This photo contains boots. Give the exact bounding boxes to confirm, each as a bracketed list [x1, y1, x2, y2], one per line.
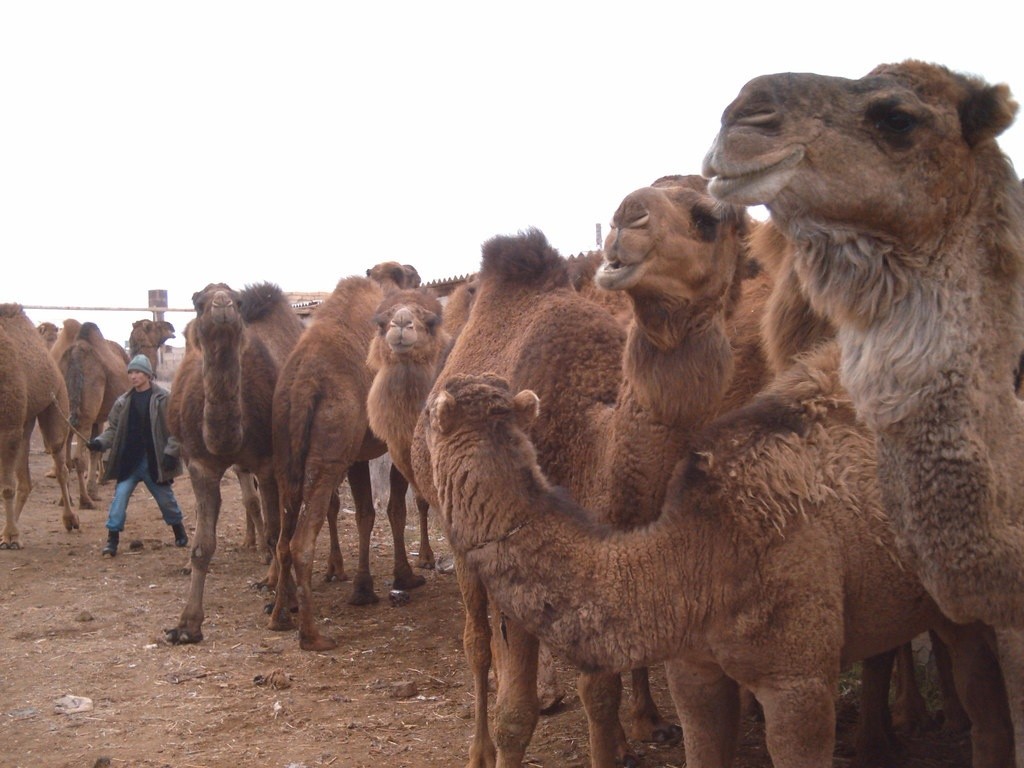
[172, 521, 188, 547]
[102, 529, 123, 557]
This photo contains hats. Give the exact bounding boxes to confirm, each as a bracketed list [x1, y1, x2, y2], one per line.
[128, 354, 153, 377]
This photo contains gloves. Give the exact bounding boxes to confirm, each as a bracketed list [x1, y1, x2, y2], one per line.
[161, 452, 177, 472]
[87, 438, 102, 451]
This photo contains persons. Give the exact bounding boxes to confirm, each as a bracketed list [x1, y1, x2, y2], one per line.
[86, 354, 188, 557]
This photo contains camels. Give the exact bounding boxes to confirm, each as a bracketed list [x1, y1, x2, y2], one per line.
[701, 57, 1024, 768]
[0, 303, 176, 552]
[411, 175, 1014, 768]
[162, 262, 480, 650]
